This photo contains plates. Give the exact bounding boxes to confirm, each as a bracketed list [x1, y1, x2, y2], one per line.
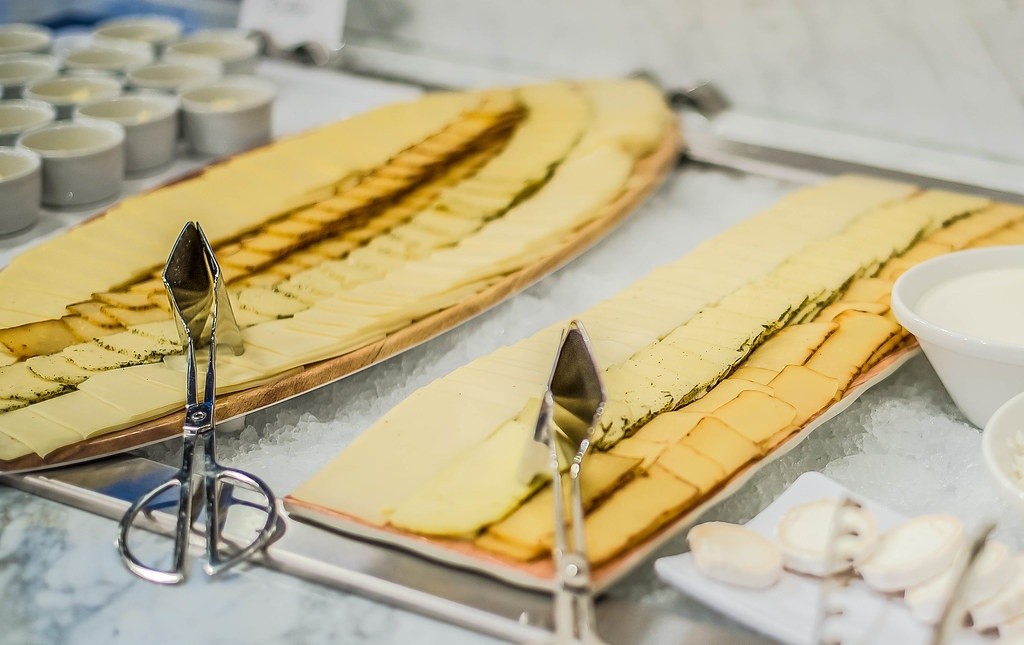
[655, 471, 1024, 645]
[284, 343, 922, 600]
[891, 245, 1024, 430]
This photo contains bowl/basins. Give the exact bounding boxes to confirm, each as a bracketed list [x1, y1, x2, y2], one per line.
[982, 391, 1024, 501]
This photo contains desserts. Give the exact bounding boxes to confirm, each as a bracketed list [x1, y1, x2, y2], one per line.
[687, 497, 1024, 645]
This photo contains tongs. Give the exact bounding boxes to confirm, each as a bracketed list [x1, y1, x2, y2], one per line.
[534, 318, 607, 645]
[116, 221, 279, 586]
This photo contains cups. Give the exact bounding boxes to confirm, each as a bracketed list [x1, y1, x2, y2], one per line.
[125, 57, 224, 95]
[0, 146, 43, 236]
[176, 76, 275, 158]
[70, 91, 179, 171]
[164, 31, 266, 76]
[21, 70, 123, 119]
[0, 113, 683, 472]
[15, 119, 127, 206]
[0, 24, 53, 59]
[0, 98, 57, 145]
[88, 17, 181, 65]
[0, 59, 63, 99]
[63, 36, 126, 81]
